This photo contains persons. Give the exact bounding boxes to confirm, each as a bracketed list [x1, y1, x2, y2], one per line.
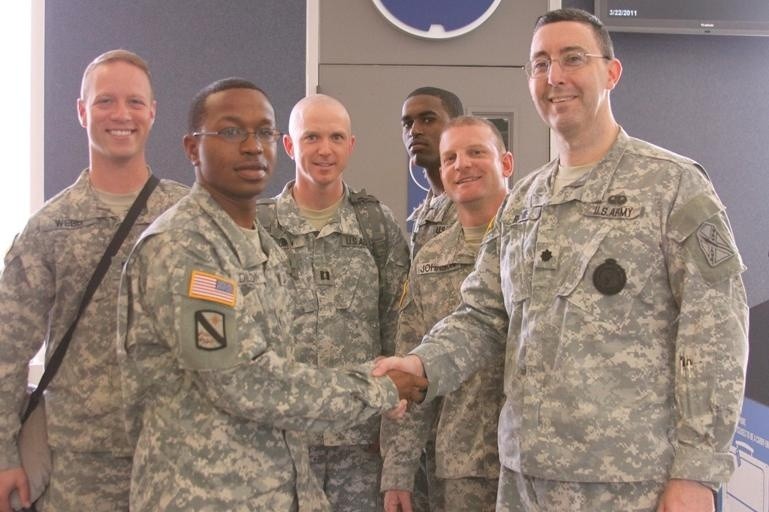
[378, 114, 515, 512]
[119, 74, 430, 511]
[255, 91, 416, 512]
[399, 84, 466, 264]
[1, 48, 191, 512]
[372, 6, 750, 512]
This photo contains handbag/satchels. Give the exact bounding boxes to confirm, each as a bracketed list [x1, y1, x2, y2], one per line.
[8, 387, 53, 512]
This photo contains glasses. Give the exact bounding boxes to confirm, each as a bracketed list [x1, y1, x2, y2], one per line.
[192, 127, 283, 145]
[521, 50, 611, 78]
